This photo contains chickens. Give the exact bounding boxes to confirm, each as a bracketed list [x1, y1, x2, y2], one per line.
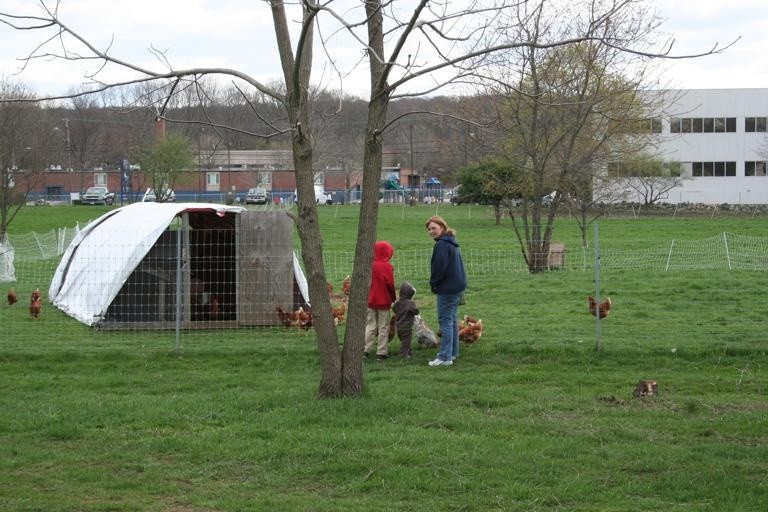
[457, 315, 483, 344]
[29, 287, 41, 318]
[326, 281, 334, 297]
[376, 314, 397, 344]
[341, 274, 352, 296]
[7, 287, 18, 305]
[587, 295, 613, 319]
[414, 312, 439, 347]
[332, 303, 346, 320]
[273, 306, 313, 337]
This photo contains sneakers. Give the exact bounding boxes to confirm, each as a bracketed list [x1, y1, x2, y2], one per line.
[363, 351, 369, 359]
[428, 357, 453, 367]
[376, 354, 389, 361]
[436, 352, 457, 361]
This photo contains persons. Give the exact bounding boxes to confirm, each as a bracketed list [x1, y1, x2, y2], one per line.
[208, 189, 459, 208]
[392, 280, 419, 358]
[424, 215, 468, 366]
[363, 242, 396, 360]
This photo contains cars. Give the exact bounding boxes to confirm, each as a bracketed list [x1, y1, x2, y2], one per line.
[146, 187, 175, 202]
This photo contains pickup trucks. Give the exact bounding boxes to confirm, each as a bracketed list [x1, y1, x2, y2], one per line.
[246, 187, 271, 203]
[83, 186, 116, 205]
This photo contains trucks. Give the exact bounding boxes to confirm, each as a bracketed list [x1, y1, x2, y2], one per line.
[294, 184, 332, 205]
